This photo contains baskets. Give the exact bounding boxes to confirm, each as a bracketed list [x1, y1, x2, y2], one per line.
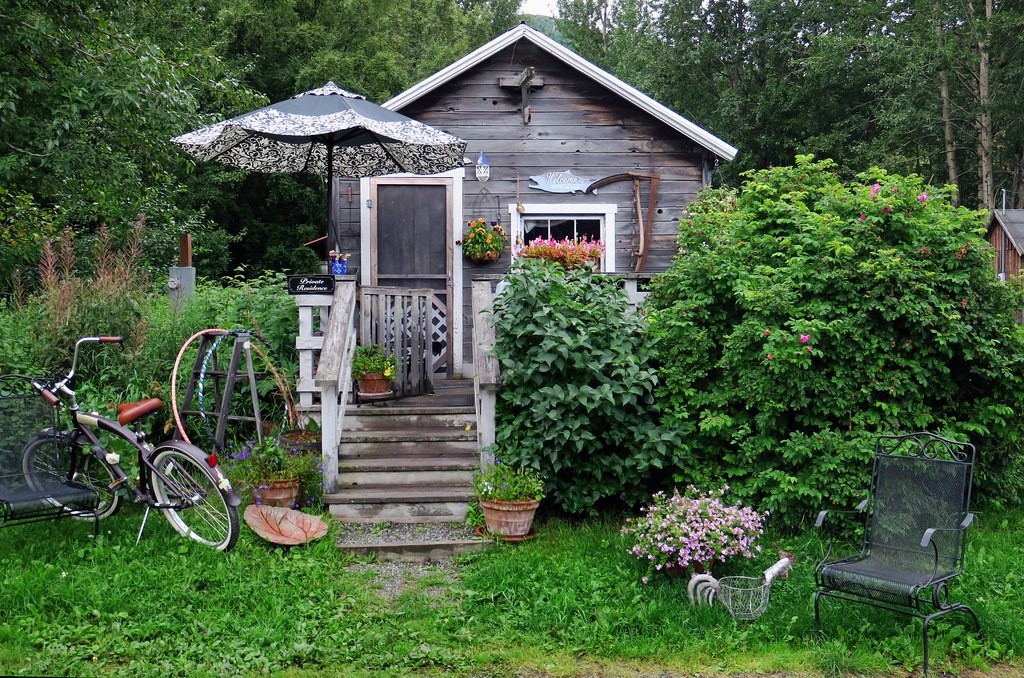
[470, 251, 500, 262]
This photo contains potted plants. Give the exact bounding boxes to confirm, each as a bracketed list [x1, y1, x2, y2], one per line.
[470, 461, 545, 542]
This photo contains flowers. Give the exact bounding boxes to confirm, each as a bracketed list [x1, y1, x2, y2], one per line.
[455, 217, 509, 261]
[225, 436, 328, 507]
[352, 344, 403, 382]
[620, 484, 771, 584]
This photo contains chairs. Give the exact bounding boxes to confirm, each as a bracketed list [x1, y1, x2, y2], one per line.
[814, 432, 985, 678]
[0, 374, 100, 548]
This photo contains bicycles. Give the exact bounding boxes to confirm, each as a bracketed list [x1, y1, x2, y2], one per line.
[20, 336, 242, 554]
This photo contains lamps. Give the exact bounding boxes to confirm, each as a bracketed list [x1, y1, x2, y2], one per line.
[475, 150, 490, 182]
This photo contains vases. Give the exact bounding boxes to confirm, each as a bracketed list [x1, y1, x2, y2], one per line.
[356, 373, 393, 399]
[247, 478, 299, 509]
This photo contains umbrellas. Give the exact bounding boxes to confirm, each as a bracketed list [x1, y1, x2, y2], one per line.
[169, 81, 474, 273]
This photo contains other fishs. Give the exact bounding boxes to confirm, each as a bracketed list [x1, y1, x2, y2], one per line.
[528, 169, 598, 195]
